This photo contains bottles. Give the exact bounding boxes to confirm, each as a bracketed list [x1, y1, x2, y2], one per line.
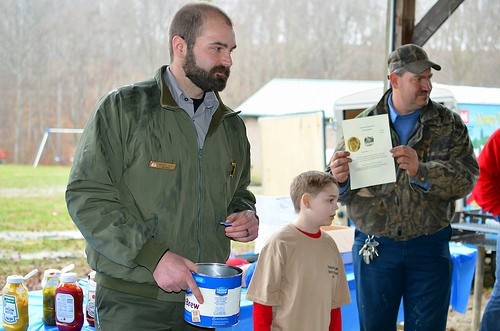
[54, 272, 84, 331]
[85, 270, 97, 326]
[42, 268, 62, 326]
[2, 274, 29, 331]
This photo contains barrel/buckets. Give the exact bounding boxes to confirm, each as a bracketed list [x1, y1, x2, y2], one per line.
[184, 262, 243, 329]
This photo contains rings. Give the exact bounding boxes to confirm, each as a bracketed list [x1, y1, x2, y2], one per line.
[245, 229, 249, 238]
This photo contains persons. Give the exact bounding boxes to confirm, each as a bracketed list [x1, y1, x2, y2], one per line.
[473, 128, 500, 331]
[65, 3, 257, 331]
[325, 41, 478, 331]
[245, 171, 352, 331]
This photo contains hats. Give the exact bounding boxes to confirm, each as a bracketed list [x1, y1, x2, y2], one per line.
[387, 44, 441, 75]
